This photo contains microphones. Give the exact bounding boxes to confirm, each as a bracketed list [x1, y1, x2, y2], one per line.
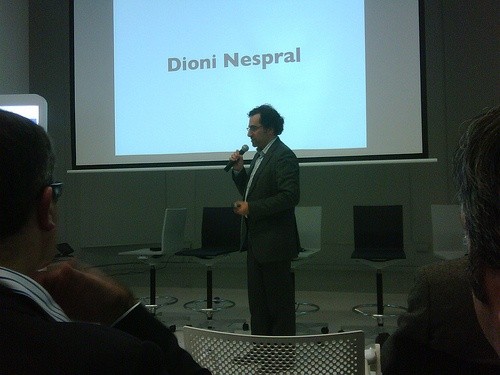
[224, 145, 249, 172]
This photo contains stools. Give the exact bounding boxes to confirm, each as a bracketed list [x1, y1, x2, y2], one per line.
[170, 205, 248, 333]
[431, 204, 467, 264]
[115, 206, 187, 319]
[350, 205, 407, 330]
[284, 205, 323, 315]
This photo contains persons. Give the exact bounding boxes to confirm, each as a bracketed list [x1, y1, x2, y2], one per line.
[232, 105, 301, 373]
[0, 109, 212, 375]
[381, 104, 500, 375]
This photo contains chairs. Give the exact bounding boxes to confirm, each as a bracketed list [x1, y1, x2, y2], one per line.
[181, 326, 370, 374]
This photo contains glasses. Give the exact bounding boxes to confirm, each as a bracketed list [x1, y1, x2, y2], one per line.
[247, 125, 262, 132]
[34, 181, 65, 203]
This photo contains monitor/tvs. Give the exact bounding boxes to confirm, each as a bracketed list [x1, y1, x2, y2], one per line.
[0, 94, 48, 132]
